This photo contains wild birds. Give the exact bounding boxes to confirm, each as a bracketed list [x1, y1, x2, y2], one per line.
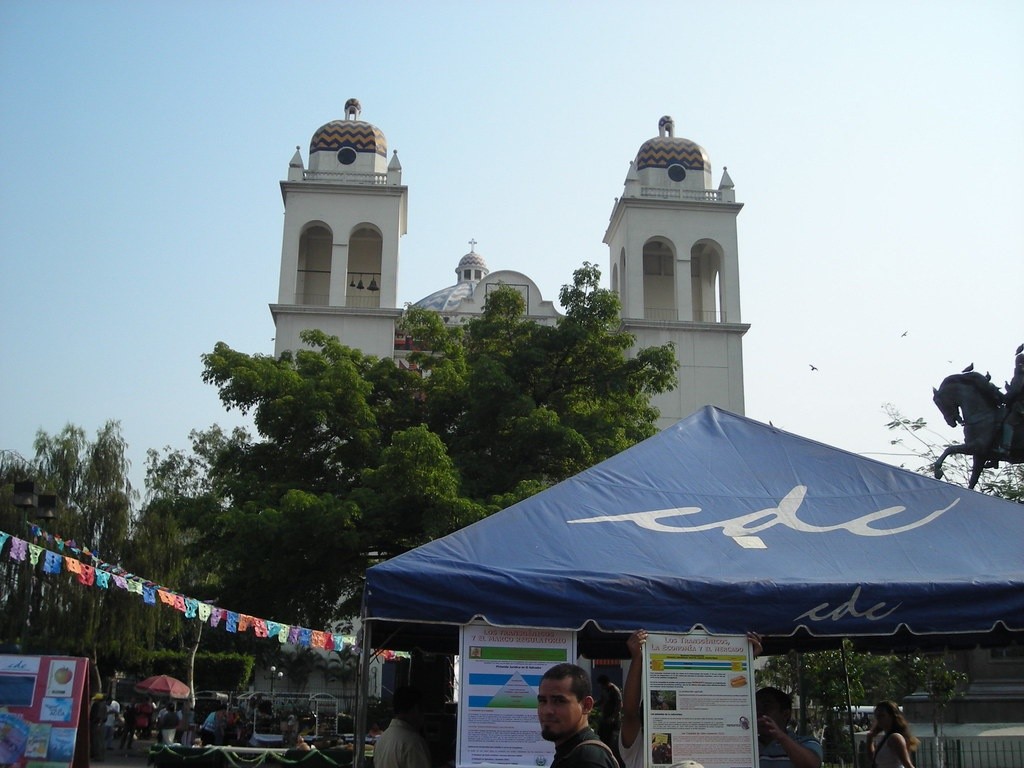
[985, 370, 991, 382]
[961, 362, 974, 373]
[808, 364, 818, 372]
[901, 330, 908, 338]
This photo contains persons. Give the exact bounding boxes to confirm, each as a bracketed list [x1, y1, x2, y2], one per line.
[756, 686, 824, 768]
[90, 692, 391, 762]
[619, 629, 762, 768]
[991, 344, 1024, 457]
[788, 710, 872, 733]
[538, 663, 618, 768]
[373, 686, 432, 768]
[866, 701, 921, 768]
[592, 676, 623, 746]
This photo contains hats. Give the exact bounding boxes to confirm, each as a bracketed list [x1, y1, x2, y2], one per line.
[92, 693, 104, 700]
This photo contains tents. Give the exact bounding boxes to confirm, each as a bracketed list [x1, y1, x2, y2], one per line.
[352, 404, 1024, 768]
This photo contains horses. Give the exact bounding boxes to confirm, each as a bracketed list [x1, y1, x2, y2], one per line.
[932, 372, 1024, 490]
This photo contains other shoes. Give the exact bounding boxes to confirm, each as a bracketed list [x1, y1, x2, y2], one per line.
[107, 747, 114, 750]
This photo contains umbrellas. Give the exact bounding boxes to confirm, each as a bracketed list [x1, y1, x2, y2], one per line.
[137, 674, 190, 698]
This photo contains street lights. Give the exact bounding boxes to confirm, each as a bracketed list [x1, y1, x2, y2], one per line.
[264, 666, 284, 698]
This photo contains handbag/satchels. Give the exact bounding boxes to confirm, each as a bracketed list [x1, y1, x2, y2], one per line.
[137, 714, 148, 727]
